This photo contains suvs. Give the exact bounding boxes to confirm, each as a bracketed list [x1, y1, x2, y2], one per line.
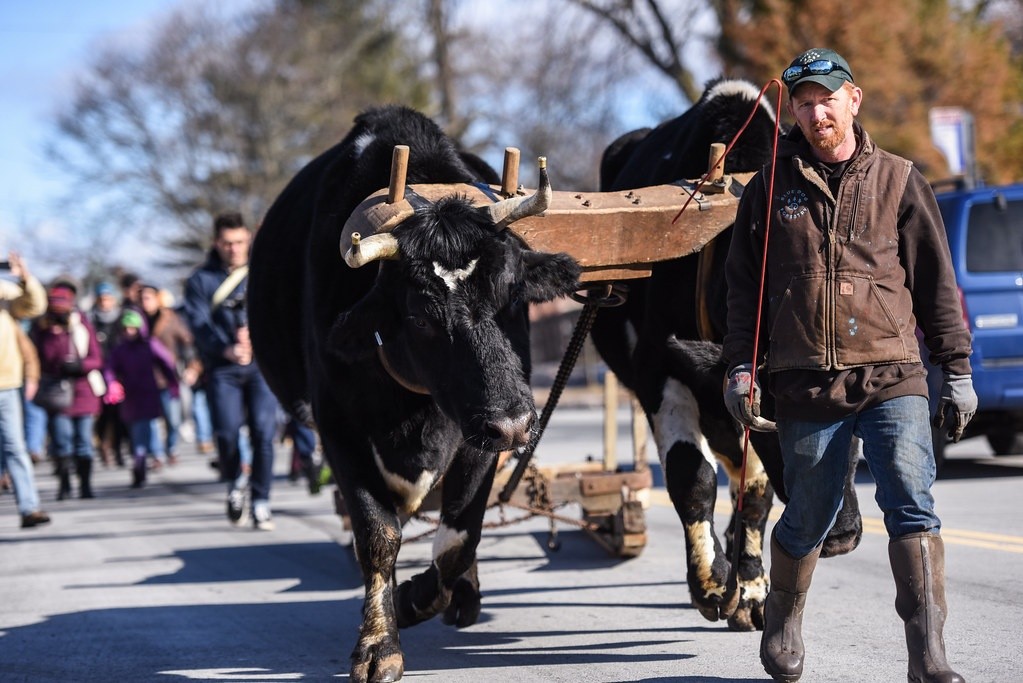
[928, 179, 1023, 457]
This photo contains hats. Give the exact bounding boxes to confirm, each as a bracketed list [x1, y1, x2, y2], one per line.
[787, 48, 854, 100]
[120, 309, 142, 328]
[137, 279, 163, 290]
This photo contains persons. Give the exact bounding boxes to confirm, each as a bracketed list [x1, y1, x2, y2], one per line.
[184, 211, 278, 531]
[719, 47, 978, 683]
[0, 251, 332, 528]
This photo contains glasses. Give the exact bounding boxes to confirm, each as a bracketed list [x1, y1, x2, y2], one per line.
[780, 59, 838, 82]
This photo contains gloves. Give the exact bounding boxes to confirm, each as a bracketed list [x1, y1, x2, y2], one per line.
[721, 363, 779, 432]
[933, 370, 978, 444]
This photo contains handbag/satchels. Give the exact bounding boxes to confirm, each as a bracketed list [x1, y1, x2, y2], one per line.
[30, 378, 74, 410]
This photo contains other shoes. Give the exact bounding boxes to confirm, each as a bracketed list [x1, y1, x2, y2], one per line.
[226, 488, 246, 522]
[131, 468, 147, 489]
[19, 511, 50, 526]
[250, 498, 277, 530]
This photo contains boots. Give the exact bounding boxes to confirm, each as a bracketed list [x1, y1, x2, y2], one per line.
[888, 532, 967, 683]
[74, 456, 96, 499]
[54, 465, 72, 501]
[759, 525, 824, 683]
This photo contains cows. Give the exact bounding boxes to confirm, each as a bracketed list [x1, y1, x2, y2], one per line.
[580, 73, 863, 635]
[244, 103, 585, 683]
